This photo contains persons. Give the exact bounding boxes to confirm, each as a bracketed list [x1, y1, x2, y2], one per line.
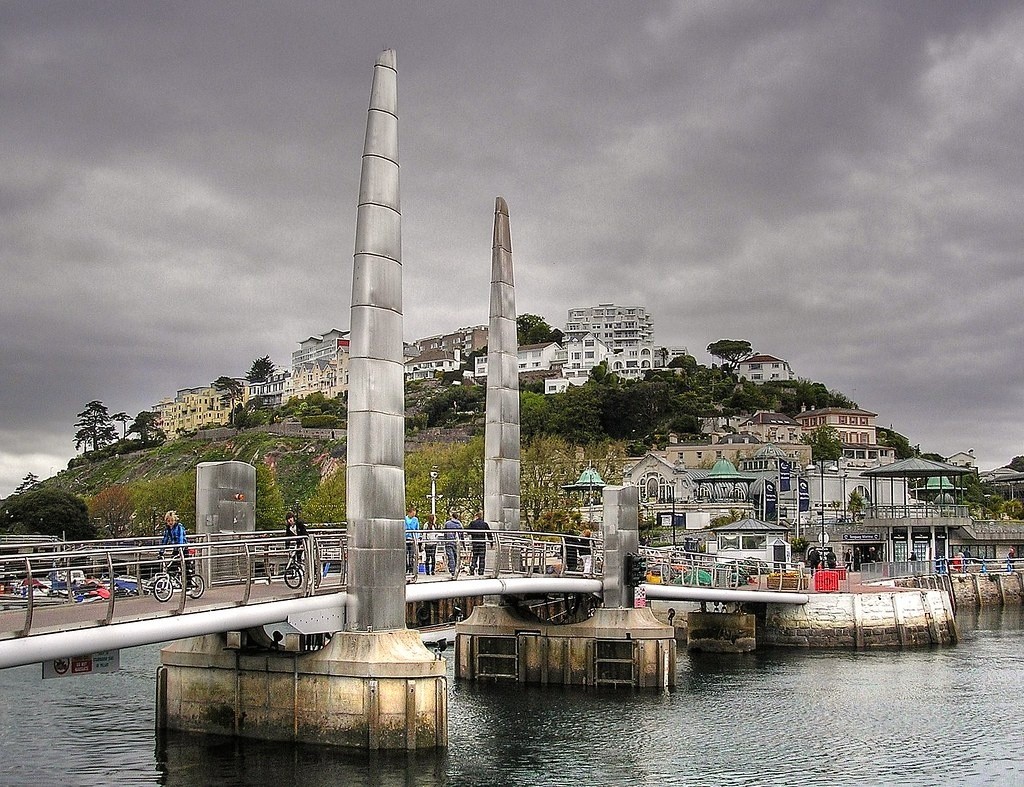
[958, 547, 971, 564]
[1009, 545, 1016, 569]
[827, 548, 836, 569]
[285, 512, 310, 564]
[467, 513, 493, 575]
[845, 549, 853, 572]
[405, 508, 420, 572]
[559, 529, 591, 571]
[445, 512, 466, 575]
[807, 547, 820, 577]
[157, 510, 192, 595]
[422, 515, 435, 575]
[908, 552, 916, 561]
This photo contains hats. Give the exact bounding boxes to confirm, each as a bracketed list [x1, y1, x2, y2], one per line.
[910, 550, 914, 552]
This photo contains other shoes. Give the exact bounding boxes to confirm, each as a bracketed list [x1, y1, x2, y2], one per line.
[186, 588, 193, 595]
[173, 580, 181, 588]
[426, 572, 435, 575]
[295, 577, 299, 580]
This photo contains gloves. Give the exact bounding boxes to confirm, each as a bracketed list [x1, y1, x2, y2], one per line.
[285, 547, 290, 553]
[157, 552, 164, 559]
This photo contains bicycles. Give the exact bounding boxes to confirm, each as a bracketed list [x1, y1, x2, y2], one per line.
[284, 544, 321, 589]
[154, 554, 205, 602]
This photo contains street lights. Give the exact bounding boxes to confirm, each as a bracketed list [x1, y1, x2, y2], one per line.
[587, 459, 597, 531]
[805, 453, 839, 568]
[669, 479, 676, 545]
[429, 466, 439, 524]
